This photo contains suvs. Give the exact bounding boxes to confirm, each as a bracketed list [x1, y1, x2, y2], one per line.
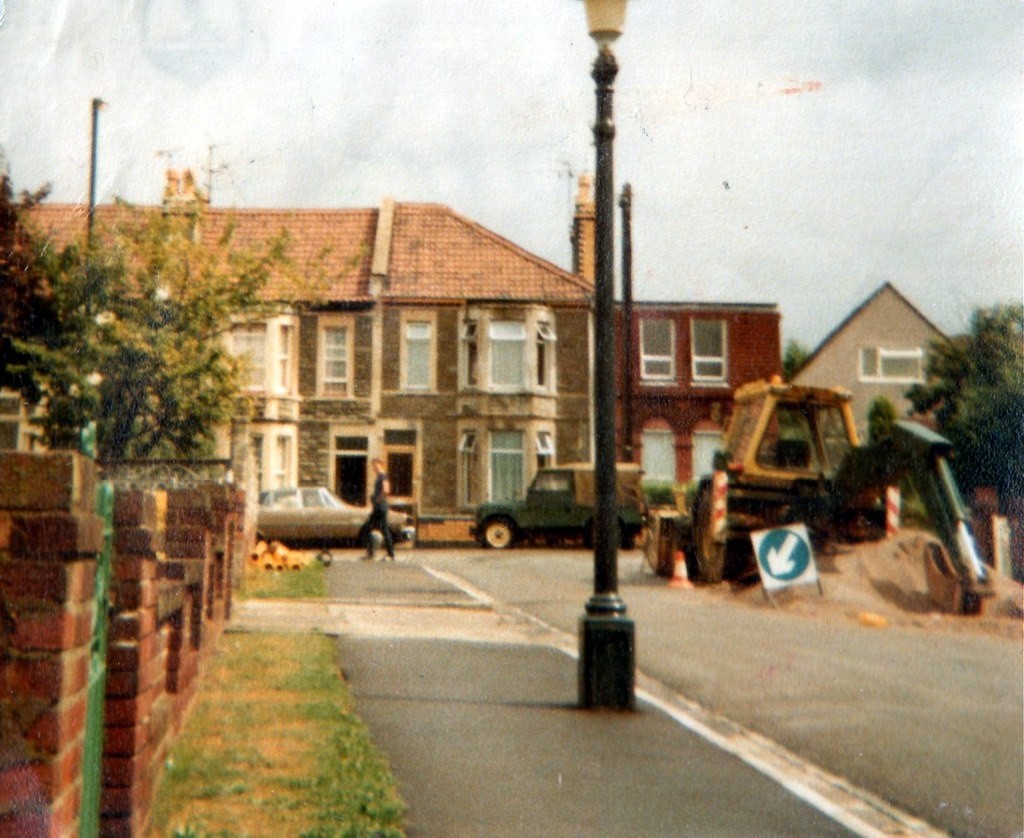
[475, 464, 648, 548]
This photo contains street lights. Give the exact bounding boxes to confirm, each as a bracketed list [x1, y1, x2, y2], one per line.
[87, 97, 107, 247]
[577, 1, 637, 717]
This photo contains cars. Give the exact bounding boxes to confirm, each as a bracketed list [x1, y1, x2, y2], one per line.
[257, 485, 415, 548]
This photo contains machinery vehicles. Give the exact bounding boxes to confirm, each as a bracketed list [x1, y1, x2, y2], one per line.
[644, 375, 1000, 615]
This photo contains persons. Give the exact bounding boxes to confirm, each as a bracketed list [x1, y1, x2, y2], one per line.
[357, 459, 396, 563]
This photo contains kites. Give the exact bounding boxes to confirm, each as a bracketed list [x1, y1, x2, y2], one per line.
[775, 77, 827, 95]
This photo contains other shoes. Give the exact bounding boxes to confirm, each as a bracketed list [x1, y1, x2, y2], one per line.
[359, 554, 375, 561]
[379, 554, 395, 561]
